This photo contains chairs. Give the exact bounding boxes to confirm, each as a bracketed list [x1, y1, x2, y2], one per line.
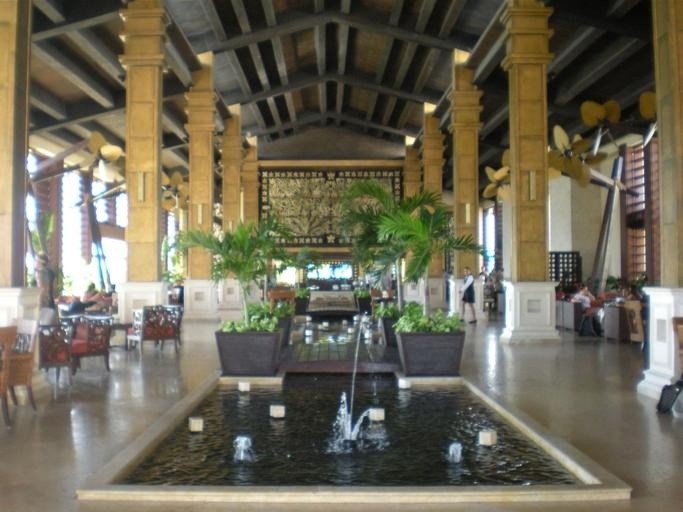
[0, 294, 115, 429]
[556, 290, 648, 351]
[127, 285, 184, 354]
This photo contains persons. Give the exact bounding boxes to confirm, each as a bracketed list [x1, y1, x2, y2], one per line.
[491, 269, 503, 313]
[622, 288, 629, 297]
[627, 285, 641, 300]
[459, 266, 476, 320]
[478, 267, 487, 278]
[569, 284, 591, 335]
[58, 279, 116, 314]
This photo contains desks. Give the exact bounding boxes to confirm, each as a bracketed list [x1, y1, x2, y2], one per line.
[110, 323, 132, 351]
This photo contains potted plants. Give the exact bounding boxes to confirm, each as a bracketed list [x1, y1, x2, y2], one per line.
[336, 178, 498, 375]
[169, 269, 186, 286]
[165, 209, 323, 375]
